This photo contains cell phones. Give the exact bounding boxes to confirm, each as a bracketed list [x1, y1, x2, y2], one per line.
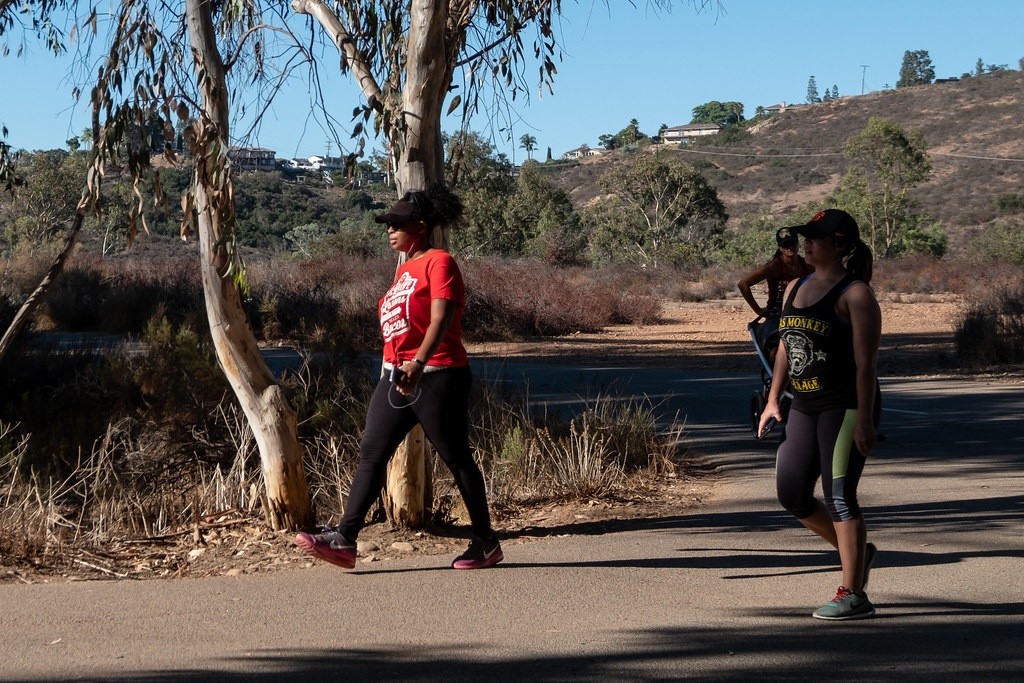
[758, 418, 776, 438]
[390, 366, 418, 397]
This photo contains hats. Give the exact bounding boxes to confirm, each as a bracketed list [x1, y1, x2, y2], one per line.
[375, 198, 428, 227]
[776, 226, 799, 246]
[788, 209, 860, 246]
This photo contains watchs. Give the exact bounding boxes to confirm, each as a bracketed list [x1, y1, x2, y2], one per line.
[412, 357, 424, 366]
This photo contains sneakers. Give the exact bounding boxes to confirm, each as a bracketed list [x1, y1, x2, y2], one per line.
[452, 529, 504, 569]
[296, 527, 356, 569]
[863, 542, 877, 589]
[813, 587, 876, 620]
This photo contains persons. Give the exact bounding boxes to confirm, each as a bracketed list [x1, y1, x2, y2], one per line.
[292, 201, 504, 569]
[739, 209, 882, 621]
